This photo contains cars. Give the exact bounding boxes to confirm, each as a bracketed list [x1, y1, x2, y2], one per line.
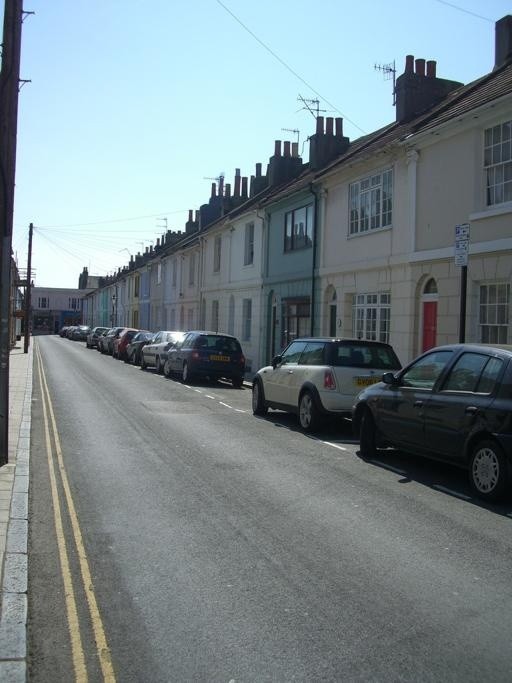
[252, 336, 403, 432]
[60, 325, 246, 387]
[352, 341, 511, 505]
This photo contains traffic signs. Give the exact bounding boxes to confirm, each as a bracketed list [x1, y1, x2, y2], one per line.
[455, 222, 470, 267]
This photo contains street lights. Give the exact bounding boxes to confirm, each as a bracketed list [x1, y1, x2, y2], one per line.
[111, 293, 116, 327]
